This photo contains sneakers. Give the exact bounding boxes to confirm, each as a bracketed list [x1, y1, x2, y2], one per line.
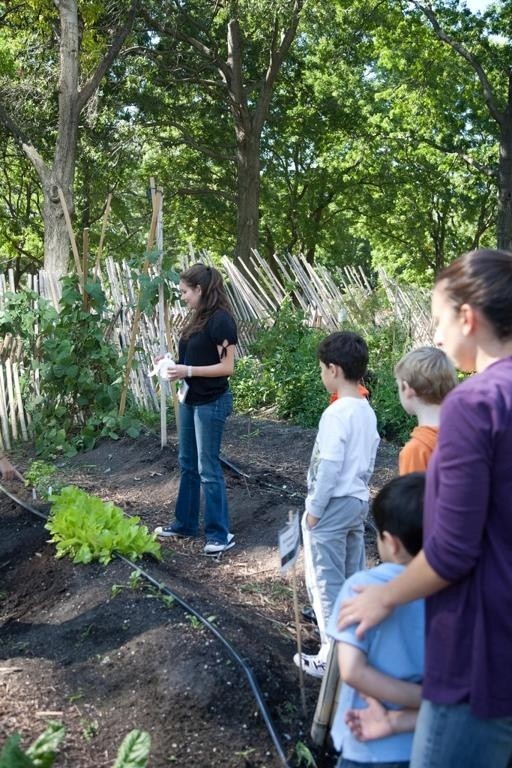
[293, 653, 328, 679]
[155, 526, 192, 538]
[301, 607, 317, 622]
[204, 540, 236, 552]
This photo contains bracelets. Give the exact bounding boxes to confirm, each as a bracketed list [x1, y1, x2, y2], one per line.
[188, 365, 192, 377]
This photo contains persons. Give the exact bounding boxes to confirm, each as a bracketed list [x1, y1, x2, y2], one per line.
[335, 249, 511, 768]
[391, 345, 458, 477]
[291, 330, 382, 683]
[320, 469, 425, 768]
[152, 262, 240, 555]
